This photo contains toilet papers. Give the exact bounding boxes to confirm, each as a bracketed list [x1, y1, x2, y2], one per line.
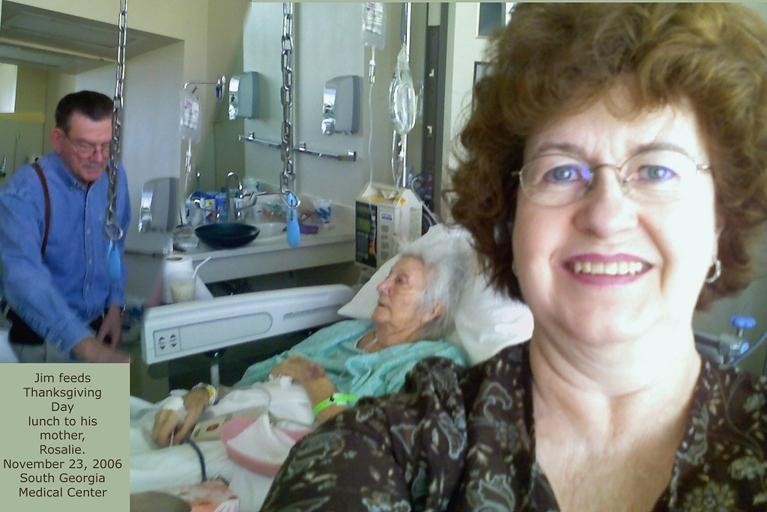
[162, 255, 193, 305]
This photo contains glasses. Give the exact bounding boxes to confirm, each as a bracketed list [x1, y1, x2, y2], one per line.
[65, 132, 111, 156]
[504, 147, 711, 204]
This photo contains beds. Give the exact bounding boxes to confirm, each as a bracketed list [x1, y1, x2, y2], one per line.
[131, 330, 740, 512]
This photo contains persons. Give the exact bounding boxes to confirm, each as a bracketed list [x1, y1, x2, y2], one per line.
[258, 2, 766, 511]
[151, 224, 479, 448]
[0, 91, 136, 363]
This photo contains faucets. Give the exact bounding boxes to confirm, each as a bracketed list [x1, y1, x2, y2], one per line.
[225, 170, 258, 221]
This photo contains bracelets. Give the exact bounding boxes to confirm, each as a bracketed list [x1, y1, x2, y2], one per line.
[312, 395, 356, 416]
[191, 382, 216, 406]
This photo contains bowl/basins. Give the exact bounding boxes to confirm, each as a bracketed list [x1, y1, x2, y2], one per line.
[194, 222, 287, 248]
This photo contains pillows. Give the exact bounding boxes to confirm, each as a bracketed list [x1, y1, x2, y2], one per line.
[335, 221, 535, 370]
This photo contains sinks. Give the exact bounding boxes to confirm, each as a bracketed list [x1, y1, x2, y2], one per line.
[248, 221, 289, 243]
